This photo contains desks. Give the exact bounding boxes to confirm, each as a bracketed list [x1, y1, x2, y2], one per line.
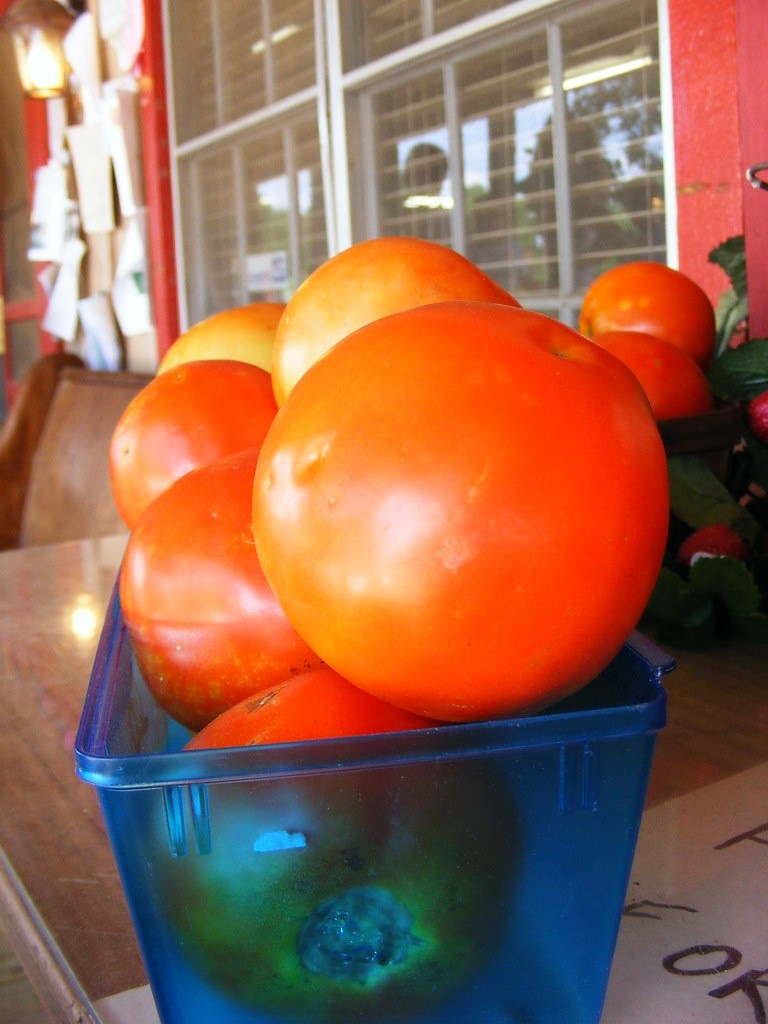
[0, 534, 768, 1024]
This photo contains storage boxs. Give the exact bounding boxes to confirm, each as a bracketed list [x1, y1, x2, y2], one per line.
[71, 591, 674, 1024]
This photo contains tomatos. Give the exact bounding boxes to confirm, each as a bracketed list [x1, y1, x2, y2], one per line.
[106, 237, 768, 1024]
